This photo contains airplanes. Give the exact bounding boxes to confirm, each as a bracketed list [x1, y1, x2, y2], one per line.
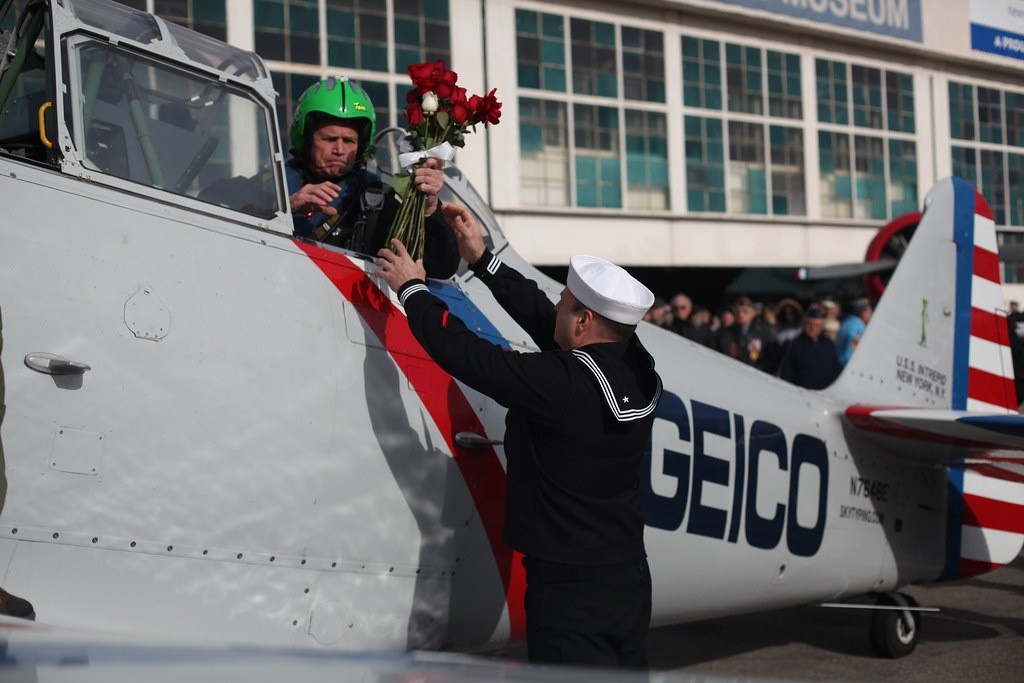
[2, 3, 1023, 680]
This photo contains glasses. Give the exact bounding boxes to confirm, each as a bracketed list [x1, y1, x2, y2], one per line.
[674, 306, 685, 310]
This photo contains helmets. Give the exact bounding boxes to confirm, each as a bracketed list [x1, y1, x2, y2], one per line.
[291, 77, 376, 166]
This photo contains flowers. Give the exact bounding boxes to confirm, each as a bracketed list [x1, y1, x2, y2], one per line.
[382, 58, 504, 259]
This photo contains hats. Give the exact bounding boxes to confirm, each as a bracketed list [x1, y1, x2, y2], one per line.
[736, 297, 754, 308]
[857, 300, 869, 309]
[1009, 298, 1020, 302]
[567, 254, 655, 325]
[807, 309, 826, 318]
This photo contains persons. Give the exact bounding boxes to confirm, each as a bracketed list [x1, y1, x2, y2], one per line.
[376, 204, 664, 683]
[1006, 301, 1024, 407]
[197, 75, 460, 281]
[642, 292, 871, 391]
